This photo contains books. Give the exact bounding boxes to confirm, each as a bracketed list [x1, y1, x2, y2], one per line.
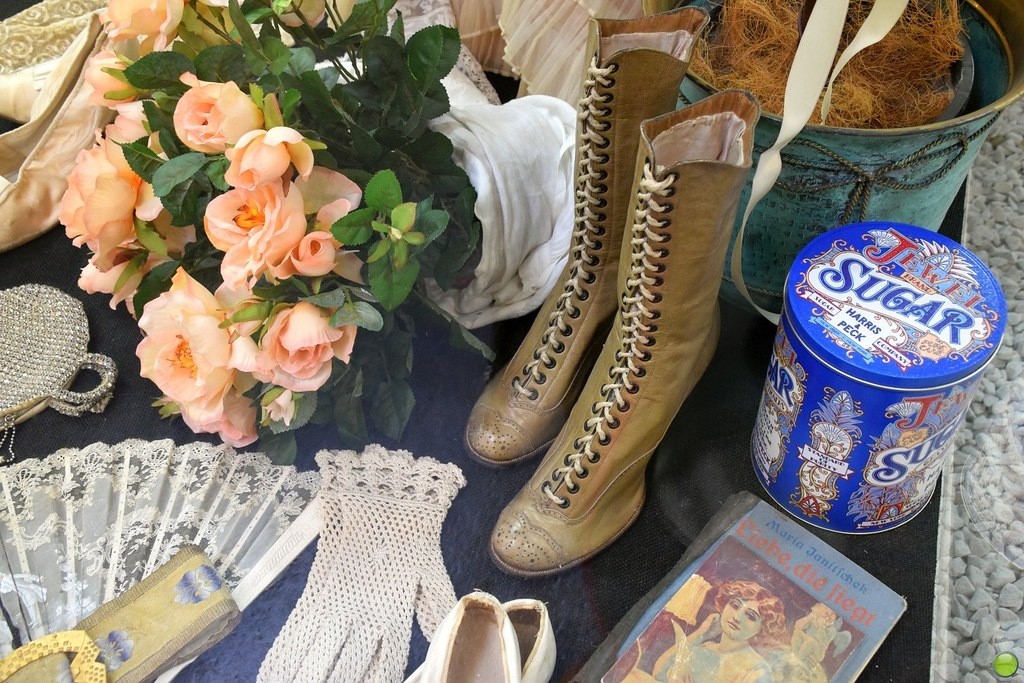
[567, 490, 907, 683]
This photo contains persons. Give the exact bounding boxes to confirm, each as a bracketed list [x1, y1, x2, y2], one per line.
[654, 578, 836, 683]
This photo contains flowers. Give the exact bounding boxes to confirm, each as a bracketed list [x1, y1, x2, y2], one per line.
[59, 0, 497, 466]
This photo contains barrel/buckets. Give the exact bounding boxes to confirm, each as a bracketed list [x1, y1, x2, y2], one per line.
[642, 0, 1024, 316]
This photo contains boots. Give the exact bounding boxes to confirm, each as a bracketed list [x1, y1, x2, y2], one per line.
[464, 6, 710, 471]
[489, 89, 761, 577]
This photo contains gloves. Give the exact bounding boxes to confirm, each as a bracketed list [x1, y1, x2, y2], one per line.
[257, 444, 468, 683]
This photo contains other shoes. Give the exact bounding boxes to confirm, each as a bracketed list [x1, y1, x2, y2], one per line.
[0, 6, 140, 251]
[403, 589, 556, 683]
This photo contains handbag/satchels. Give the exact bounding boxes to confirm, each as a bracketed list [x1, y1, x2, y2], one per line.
[0, 284, 118, 432]
